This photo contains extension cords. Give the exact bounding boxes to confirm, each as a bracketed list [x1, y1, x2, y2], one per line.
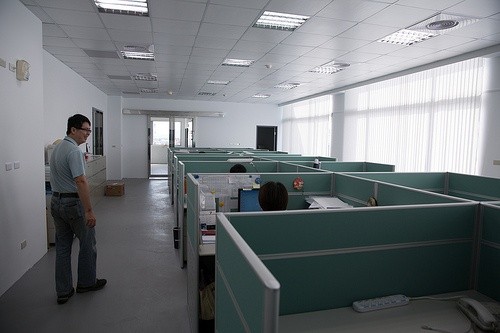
[352, 294, 409, 313]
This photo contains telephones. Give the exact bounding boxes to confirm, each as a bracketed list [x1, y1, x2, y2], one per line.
[86, 143, 91, 152]
[456, 297, 500, 331]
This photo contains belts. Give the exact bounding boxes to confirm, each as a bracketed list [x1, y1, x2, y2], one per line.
[53, 191, 79, 197]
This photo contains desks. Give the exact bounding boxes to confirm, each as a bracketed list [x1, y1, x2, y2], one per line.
[200, 229, 216, 255]
[46, 155, 107, 243]
[278, 289, 500, 333]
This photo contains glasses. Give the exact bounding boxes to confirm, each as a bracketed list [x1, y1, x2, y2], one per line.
[80, 127, 91, 132]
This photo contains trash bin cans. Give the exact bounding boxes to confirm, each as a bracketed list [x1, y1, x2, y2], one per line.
[173, 226, 180, 249]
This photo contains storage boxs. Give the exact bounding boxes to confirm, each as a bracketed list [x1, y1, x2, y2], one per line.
[104, 183, 125, 197]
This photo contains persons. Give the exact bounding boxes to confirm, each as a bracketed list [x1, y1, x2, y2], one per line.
[51, 114, 108, 305]
[229, 164, 247, 173]
[258, 181, 289, 212]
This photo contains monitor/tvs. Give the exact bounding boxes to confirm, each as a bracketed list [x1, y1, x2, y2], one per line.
[238, 188, 263, 212]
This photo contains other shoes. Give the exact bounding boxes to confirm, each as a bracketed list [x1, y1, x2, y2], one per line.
[76, 278, 107, 293]
[57, 287, 74, 304]
